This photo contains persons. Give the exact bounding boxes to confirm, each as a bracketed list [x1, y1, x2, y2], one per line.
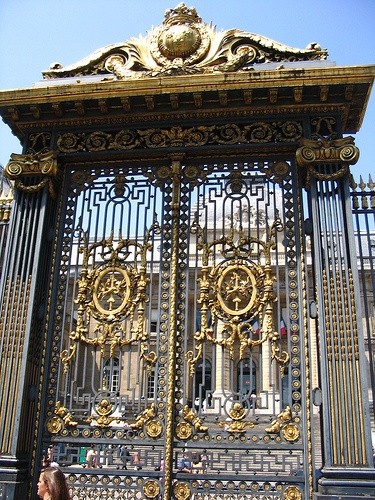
[45, 443, 207, 475]
[36, 466, 72, 500]
